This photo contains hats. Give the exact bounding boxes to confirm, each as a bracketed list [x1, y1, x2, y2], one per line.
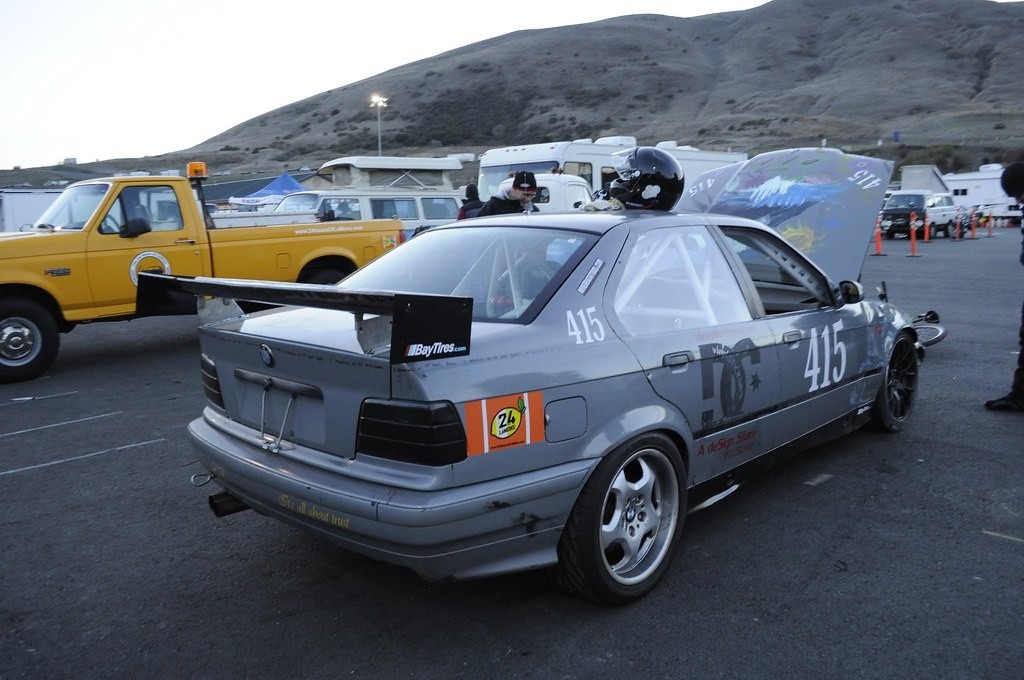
[466, 184, 479, 197]
[513, 171, 538, 192]
[1001, 163, 1024, 197]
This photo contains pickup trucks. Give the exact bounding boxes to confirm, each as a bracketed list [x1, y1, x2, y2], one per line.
[0, 173, 408, 385]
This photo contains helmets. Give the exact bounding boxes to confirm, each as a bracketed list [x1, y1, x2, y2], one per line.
[611, 146, 685, 210]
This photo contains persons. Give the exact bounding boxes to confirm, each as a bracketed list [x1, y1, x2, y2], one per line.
[457, 184, 485, 221]
[476, 171, 540, 218]
[983, 162, 1024, 414]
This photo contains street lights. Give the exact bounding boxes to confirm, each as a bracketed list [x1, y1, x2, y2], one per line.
[369, 95, 389, 156]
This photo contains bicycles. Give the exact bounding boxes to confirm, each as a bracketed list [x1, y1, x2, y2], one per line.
[873, 280, 947, 347]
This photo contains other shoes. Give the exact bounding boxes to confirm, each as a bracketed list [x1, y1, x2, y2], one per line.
[985, 395, 1024, 412]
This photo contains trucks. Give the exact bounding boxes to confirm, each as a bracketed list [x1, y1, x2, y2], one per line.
[476, 134, 749, 207]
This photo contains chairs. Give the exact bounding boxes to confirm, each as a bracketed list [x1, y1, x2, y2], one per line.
[325, 199, 451, 220]
[135, 204, 152, 223]
[521, 261, 566, 303]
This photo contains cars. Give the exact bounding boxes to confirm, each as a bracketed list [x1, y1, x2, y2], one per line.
[134, 145, 925, 610]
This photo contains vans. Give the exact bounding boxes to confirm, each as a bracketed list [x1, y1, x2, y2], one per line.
[872, 181, 969, 241]
[217, 134, 596, 245]
